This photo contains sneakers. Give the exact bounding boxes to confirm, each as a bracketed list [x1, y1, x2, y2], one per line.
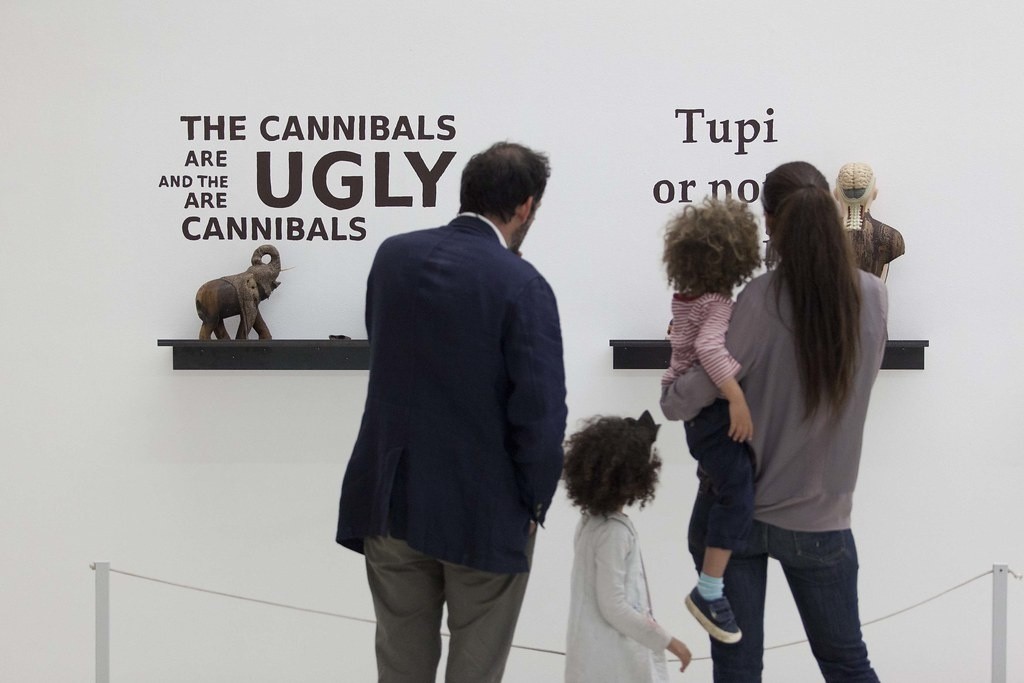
[685, 586, 743, 645]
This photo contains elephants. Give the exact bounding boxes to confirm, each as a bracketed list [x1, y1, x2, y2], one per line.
[195, 245, 281, 340]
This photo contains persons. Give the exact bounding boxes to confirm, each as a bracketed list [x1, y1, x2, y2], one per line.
[560, 413, 691, 683]
[658, 159, 880, 683]
[334, 141, 568, 683]
[831, 162, 905, 286]
[660, 194, 757, 644]
